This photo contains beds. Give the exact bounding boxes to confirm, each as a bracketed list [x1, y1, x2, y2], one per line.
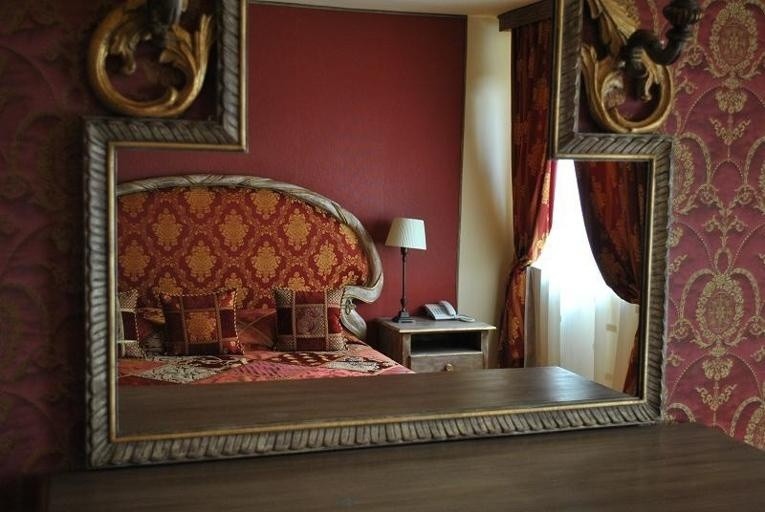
[114, 172, 416, 387]
[117, 288, 148, 360]
[158, 288, 246, 357]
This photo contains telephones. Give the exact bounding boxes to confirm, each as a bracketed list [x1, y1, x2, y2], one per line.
[425, 300, 475, 322]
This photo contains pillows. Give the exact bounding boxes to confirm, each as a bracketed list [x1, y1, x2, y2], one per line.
[272, 286, 349, 352]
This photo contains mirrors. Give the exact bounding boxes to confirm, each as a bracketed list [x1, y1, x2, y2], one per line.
[82, 0, 676, 468]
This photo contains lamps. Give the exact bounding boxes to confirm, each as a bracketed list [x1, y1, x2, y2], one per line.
[385, 216, 427, 324]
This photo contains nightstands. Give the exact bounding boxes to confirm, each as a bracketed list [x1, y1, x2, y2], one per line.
[377, 315, 497, 374]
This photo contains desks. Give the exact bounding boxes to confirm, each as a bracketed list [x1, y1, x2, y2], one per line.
[40, 421, 765, 512]
